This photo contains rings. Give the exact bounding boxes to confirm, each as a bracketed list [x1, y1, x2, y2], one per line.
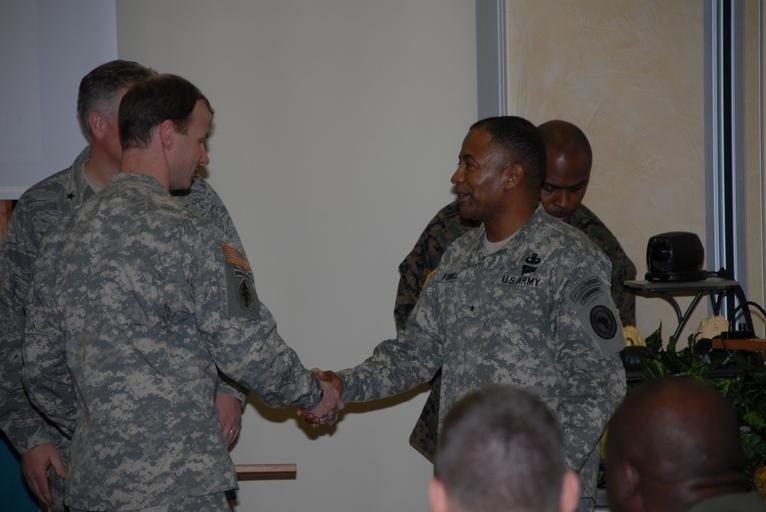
[318, 416, 328, 425]
[229, 429, 236, 434]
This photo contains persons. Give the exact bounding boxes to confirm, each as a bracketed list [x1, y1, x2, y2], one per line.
[602, 375, 766, 512]
[393, 118, 638, 472]
[20, 72, 346, 512]
[0, 57, 259, 512]
[295, 113, 629, 512]
[426, 384, 583, 512]
